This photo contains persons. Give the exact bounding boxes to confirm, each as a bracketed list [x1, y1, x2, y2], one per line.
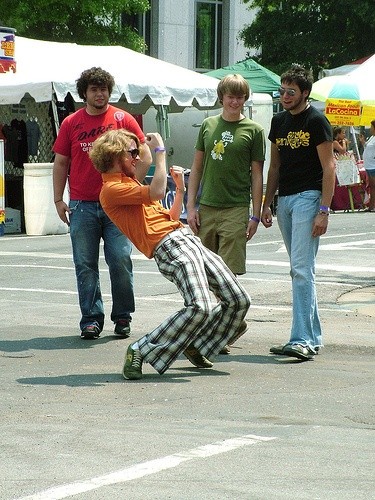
[333, 127, 348, 154]
[186, 74, 266, 354]
[359, 121, 375, 212]
[89, 128, 251, 380]
[261, 67, 336, 359]
[53, 66, 152, 338]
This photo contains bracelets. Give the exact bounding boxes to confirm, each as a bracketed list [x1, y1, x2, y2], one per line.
[318, 206, 330, 216]
[154, 147, 165, 152]
[251, 217, 259, 223]
[176, 187, 186, 193]
[55, 200, 62, 204]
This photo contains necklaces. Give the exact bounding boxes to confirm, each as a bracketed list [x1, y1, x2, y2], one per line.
[302, 104, 308, 111]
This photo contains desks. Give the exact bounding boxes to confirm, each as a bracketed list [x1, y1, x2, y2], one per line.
[145, 175, 202, 213]
[330, 170, 370, 213]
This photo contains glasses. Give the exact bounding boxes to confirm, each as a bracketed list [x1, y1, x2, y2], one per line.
[127, 149, 139, 158]
[278, 87, 301, 96]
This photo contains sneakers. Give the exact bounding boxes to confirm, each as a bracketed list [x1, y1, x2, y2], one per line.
[227, 320, 249, 345]
[269, 344, 284, 354]
[122, 345, 143, 381]
[283, 343, 314, 361]
[81, 326, 100, 339]
[184, 344, 214, 368]
[114, 321, 131, 337]
[219, 345, 230, 354]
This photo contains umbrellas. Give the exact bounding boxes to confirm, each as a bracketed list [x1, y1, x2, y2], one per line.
[308, 74, 375, 159]
[204, 59, 281, 120]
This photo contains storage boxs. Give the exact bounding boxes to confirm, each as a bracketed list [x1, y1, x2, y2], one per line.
[4, 207, 21, 233]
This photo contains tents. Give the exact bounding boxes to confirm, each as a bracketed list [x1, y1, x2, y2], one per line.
[0, 36, 254, 140]
